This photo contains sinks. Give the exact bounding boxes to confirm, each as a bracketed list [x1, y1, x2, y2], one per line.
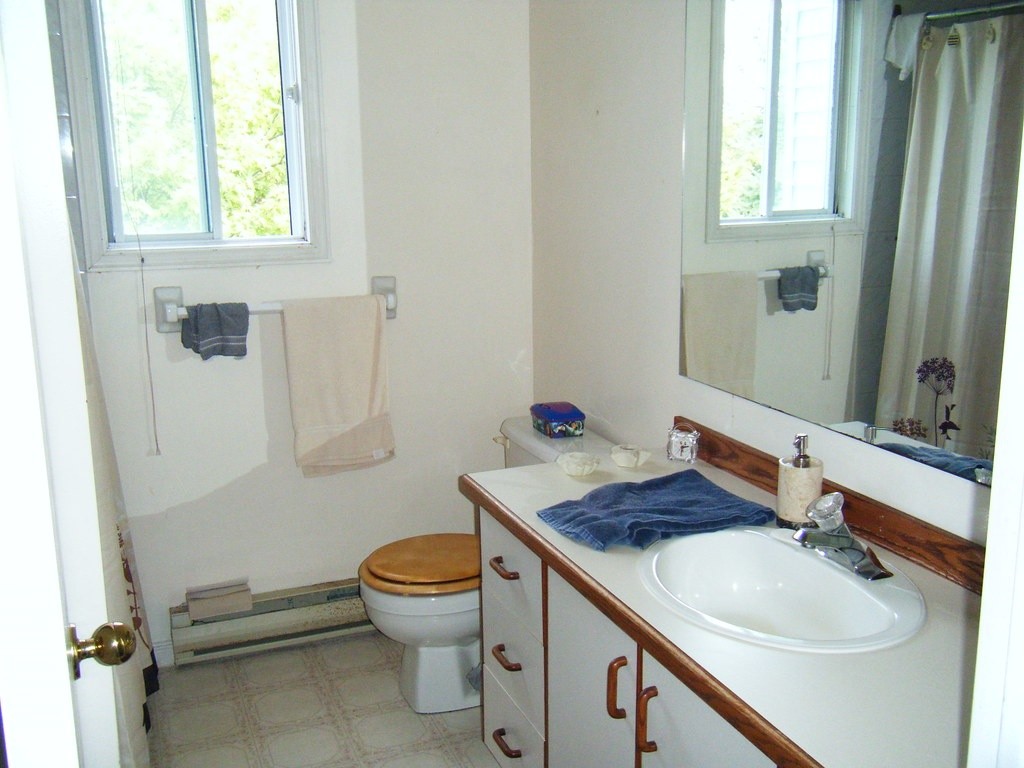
[638, 523, 928, 654]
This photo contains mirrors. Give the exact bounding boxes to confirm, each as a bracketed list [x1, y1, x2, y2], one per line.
[677, 1, 1024, 488]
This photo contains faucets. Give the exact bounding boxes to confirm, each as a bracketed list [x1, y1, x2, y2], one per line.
[791, 491, 894, 581]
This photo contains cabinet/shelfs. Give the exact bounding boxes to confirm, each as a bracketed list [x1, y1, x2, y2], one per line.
[545, 566, 778, 768]
[472, 505, 548, 767]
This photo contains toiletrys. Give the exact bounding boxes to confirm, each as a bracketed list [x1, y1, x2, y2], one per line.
[776, 433, 824, 531]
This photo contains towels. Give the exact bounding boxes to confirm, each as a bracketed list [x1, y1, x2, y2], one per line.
[180, 293, 400, 479]
[534, 468, 776, 552]
[875, 443, 993, 489]
[679, 266, 821, 403]
[882, 10, 935, 82]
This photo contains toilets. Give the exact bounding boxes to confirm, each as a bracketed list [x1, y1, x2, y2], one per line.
[357, 412, 632, 715]
